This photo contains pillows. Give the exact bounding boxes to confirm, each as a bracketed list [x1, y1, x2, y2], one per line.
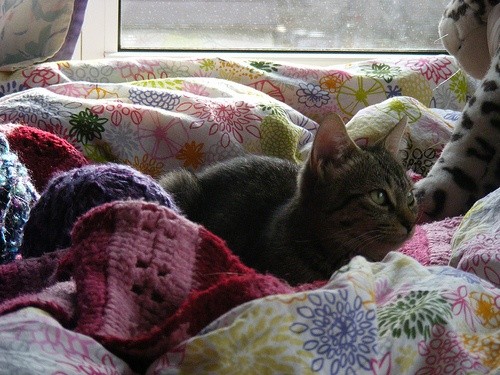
[0, 0, 88, 71]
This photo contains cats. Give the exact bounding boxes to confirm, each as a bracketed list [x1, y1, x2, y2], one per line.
[154, 111, 421, 288]
